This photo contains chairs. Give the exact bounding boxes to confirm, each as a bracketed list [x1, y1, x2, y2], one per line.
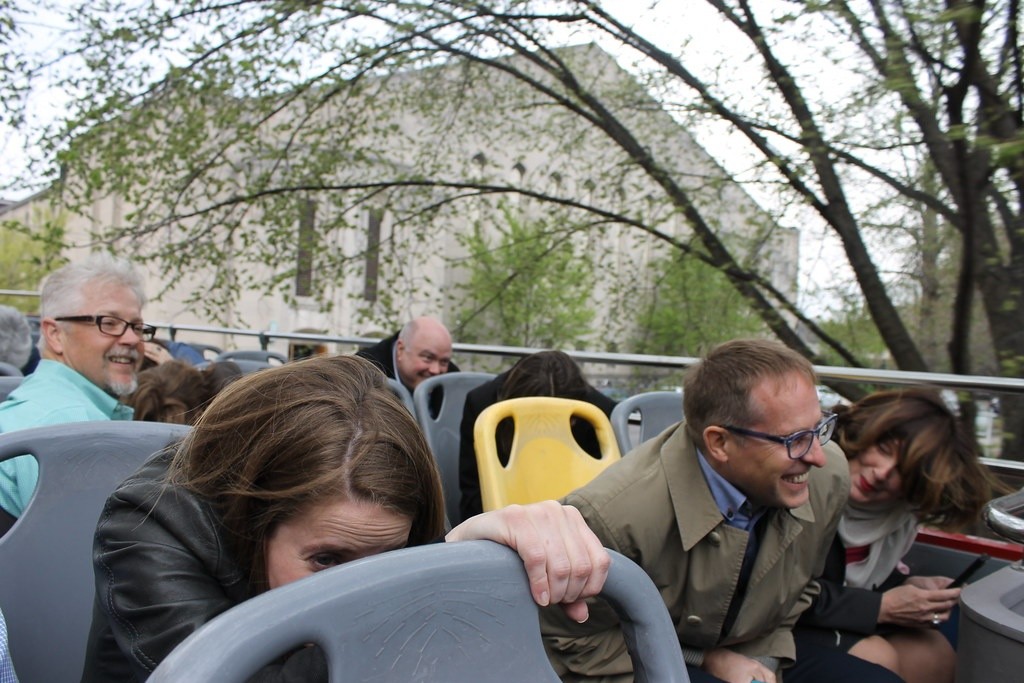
[1, 316, 690, 683]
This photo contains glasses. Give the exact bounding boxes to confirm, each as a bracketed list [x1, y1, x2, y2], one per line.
[54, 315, 158, 342]
[718, 409, 838, 460]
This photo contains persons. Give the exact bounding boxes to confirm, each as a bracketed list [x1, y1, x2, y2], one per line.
[356, 316, 460, 419]
[0, 254, 156, 520]
[545, 336, 908, 683]
[458, 348, 619, 526]
[0, 305, 41, 376]
[124, 360, 242, 427]
[794, 387, 996, 683]
[80, 354, 610, 683]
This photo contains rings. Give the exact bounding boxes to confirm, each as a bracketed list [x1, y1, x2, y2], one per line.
[932, 613, 940, 624]
